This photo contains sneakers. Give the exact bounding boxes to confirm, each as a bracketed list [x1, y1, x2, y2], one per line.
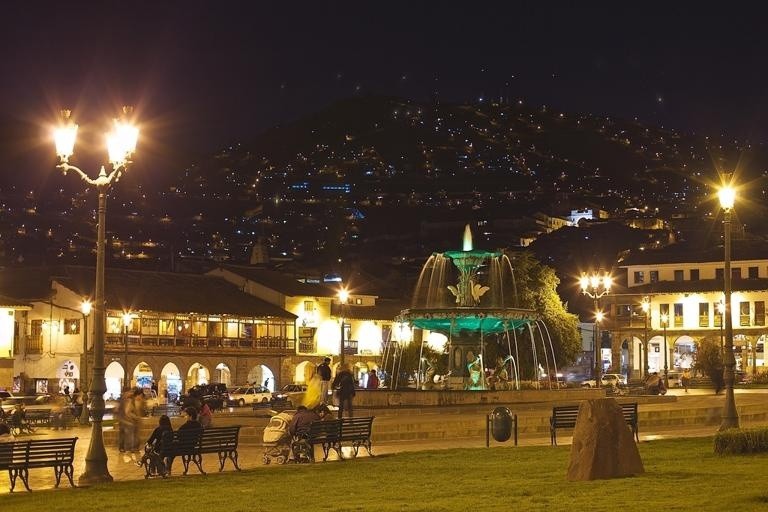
[134, 460, 142, 467]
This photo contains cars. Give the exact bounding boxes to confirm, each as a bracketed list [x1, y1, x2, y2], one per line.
[556, 370, 754, 400]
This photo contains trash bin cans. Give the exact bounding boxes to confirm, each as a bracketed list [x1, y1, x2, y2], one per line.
[492, 407, 512, 442]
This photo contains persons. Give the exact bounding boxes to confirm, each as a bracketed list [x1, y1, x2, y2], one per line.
[247, 356, 380, 465]
[681, 368, 691, 392]
[0, 399, 35, 435]
[117, 379, 228, 477]
[648, 372, 667, 397]
[64, 386, 80, 406]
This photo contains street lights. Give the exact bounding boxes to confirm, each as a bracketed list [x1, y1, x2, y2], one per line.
[52, 106, 140, 489]
[337, 289, 349, 370]
[718, 185, 741, 430]
[641, 300, 650, 377]
[662, 314, 669, 388]
[579, 264, 613, 388]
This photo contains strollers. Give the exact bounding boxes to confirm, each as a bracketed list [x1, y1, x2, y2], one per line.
[262, 410, 300, 464]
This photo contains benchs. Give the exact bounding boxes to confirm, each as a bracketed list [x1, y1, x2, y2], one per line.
[291, 416, 375, 464]
[144, 425, 243, 480]
[550, 401, 639, 449]
[0, 437, 79, 493]
[0, 409, 51, 433]
